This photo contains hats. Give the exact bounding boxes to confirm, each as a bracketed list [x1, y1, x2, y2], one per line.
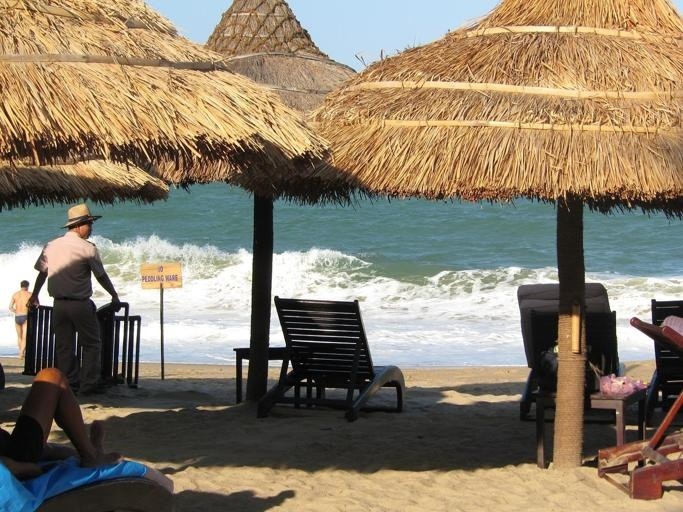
[59, 203, 103, 229]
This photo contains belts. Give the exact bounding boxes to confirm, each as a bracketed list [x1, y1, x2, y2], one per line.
[53, 296, 88, 303]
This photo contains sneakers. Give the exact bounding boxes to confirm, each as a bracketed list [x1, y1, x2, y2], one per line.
[80, 384, 110, 395]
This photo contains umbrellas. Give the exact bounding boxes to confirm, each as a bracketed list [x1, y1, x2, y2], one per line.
[199, 0, 365, 400]
[0, 152, 171, 212]
[299, 0, 681, 471]
[0, 1, 345, 218]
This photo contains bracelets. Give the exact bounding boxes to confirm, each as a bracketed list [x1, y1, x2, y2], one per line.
[111, 293, 118, 297]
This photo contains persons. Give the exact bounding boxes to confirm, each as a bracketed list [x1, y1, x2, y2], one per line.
[25, 204, 121, 396]
[8, 279, 40, 360]
[0, 362, 122, 482]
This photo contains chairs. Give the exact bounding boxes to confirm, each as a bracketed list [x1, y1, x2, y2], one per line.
[517, 282, 682, 427]
[255, 295, 406, 423]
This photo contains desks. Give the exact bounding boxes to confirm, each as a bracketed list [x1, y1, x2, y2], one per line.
[530, 384, 652, 471]
[232, 347, 313, 411]
